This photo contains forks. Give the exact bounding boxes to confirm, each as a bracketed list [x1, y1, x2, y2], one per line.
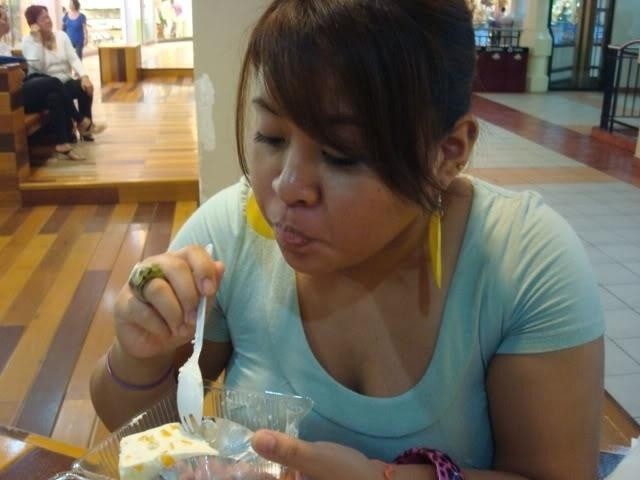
[169, 244, 212, 433]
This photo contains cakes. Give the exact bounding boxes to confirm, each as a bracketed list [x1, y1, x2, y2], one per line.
[118, 421, 218, 479]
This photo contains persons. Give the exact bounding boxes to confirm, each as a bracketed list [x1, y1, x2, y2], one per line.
[22, 3, 97, 144]
[0, 3, 106, 163]
[90, 0, 612, 480]
[61, 0, 92, 68]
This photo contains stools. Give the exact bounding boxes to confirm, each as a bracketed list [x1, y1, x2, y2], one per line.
[97, 44, 141, 89]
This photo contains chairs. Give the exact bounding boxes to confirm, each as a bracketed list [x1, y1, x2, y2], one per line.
[0, 62, 57, 177]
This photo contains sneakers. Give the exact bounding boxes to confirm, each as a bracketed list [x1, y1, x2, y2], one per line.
[52, 116, 109, 164]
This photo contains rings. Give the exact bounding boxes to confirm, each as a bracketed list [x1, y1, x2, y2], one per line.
[126, 259, 172, 307]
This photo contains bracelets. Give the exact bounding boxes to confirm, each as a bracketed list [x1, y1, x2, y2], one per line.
[103, 352, 177, 392]
[371, 457, 396, 479]
[394, 445, 464, 479]
